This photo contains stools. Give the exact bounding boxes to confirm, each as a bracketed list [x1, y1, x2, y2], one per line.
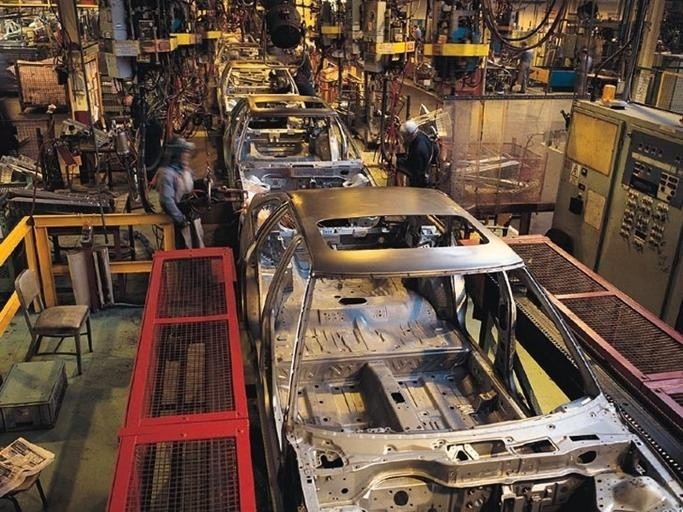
[1, 471, 49, 512]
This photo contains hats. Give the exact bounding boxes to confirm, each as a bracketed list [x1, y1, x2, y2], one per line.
[166, 137, 195, 150]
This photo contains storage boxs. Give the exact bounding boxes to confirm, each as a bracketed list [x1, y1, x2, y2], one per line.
[0, 360, 69, 431]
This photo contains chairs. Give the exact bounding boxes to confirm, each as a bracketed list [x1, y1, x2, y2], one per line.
[15, 268, 93, 374]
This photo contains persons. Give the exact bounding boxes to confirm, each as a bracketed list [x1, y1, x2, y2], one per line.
[400, 120, 435, 188]
[153, 135, 207, 250]
